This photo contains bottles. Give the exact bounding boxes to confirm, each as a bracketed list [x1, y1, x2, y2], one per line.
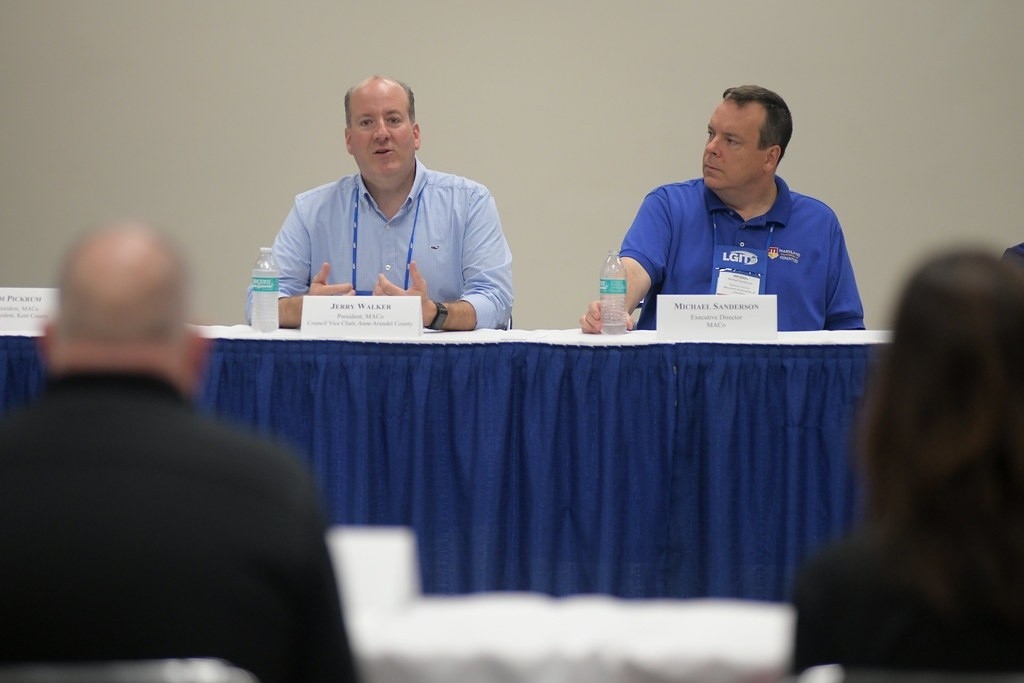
[598, 249, 628, 335]
[251, 247, 279, 333]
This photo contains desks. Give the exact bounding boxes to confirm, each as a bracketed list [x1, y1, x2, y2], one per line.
[0, 333, 1024, 607]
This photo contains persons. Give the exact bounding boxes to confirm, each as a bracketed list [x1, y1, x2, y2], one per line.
[790, 240, 1024, 683]
[0, 222, 353, 683]
[580, 85, 867, 333]
[246, 75, 514, 331]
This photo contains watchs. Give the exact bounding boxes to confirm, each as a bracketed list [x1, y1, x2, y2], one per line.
[424, 301, 448, 330]
